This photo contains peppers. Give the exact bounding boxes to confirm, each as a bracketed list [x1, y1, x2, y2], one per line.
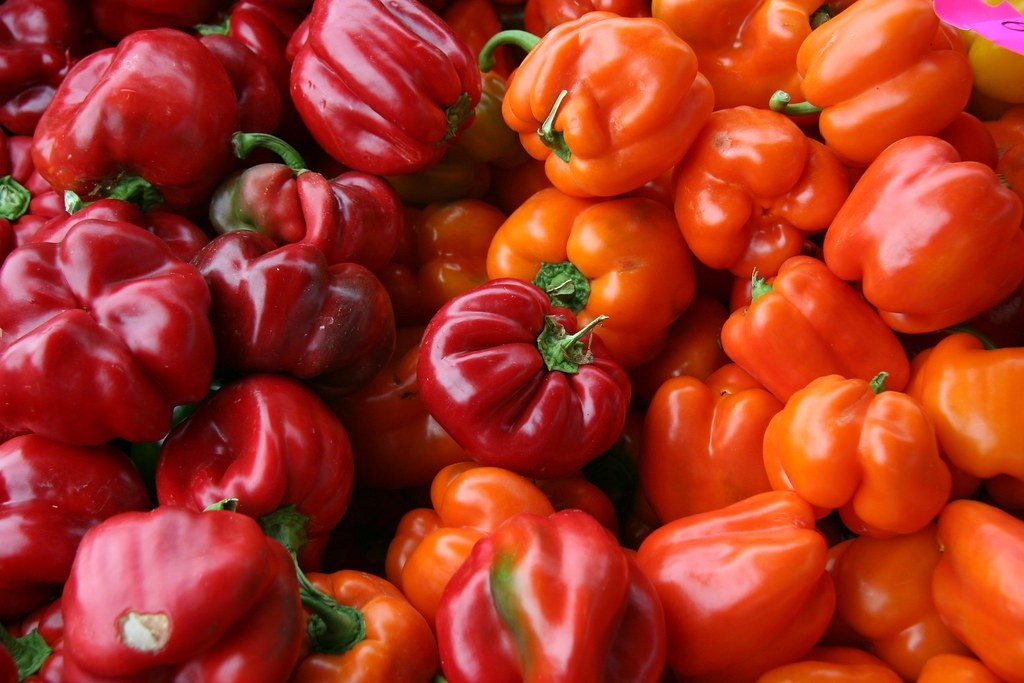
[0, 0, 1024, 683]
